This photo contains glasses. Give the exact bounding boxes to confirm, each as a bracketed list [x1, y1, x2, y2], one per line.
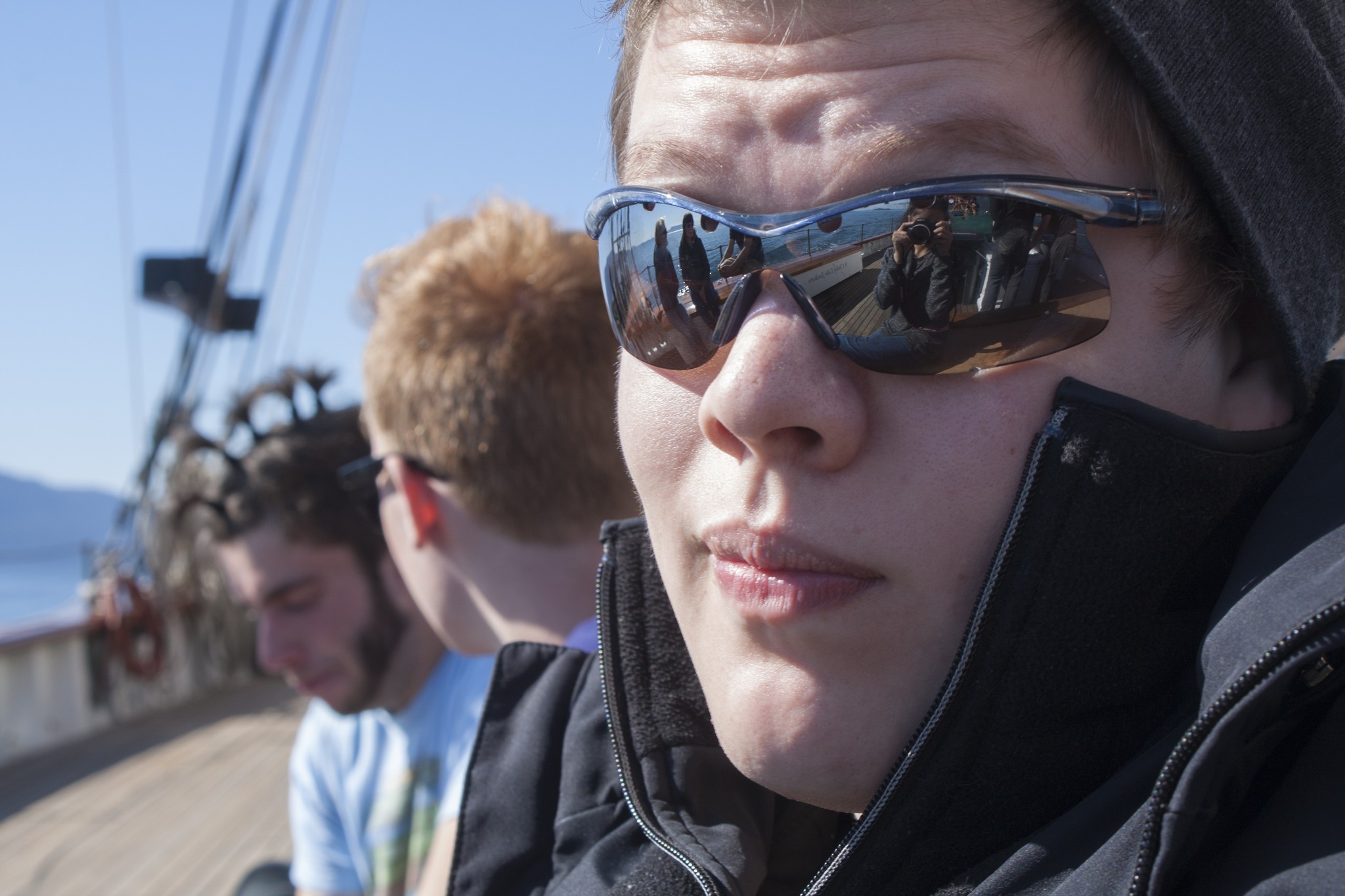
[584, 173, 1174, 375]
[335, 451, 452, 506]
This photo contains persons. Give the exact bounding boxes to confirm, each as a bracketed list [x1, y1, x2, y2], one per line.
[871, 190, 1076, 338]
[595, 1, 1345, 896]
[204, 203, 625, 896]
[651, 211, 764, 364]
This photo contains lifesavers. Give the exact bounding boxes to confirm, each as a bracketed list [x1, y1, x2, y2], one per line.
[97, 571, 166, 683]
